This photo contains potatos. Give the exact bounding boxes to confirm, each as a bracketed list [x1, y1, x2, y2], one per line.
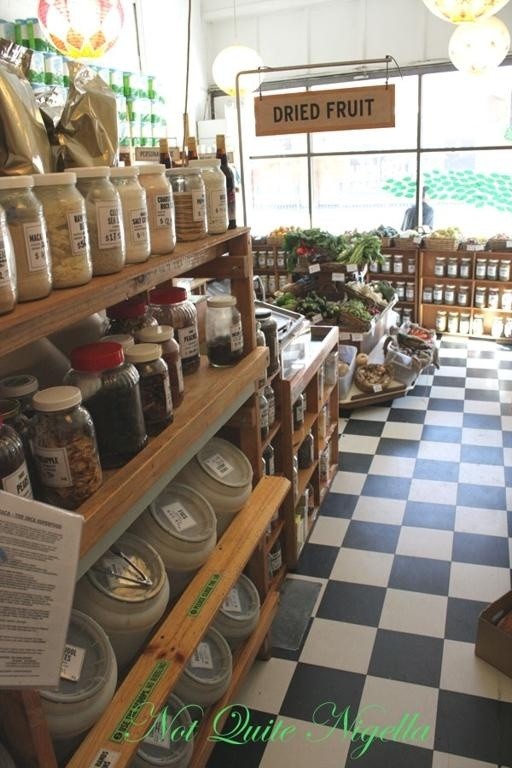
[338, 363, 348, 375]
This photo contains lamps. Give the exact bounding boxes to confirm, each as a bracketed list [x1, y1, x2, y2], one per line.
[35, 0, 125, 60]
[424, 0, 511, 79]
[211, 2, 263, 99]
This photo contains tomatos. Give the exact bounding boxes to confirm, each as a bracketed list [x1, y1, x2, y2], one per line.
[296, 238, 319, 256]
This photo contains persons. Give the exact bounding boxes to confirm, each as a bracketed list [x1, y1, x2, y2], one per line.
[401, 186, 433, 231]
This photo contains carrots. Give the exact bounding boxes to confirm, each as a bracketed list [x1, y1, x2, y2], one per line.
[408, 326, 429, 341]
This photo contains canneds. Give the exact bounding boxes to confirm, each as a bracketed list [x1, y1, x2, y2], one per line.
[0, 287, 244, 509]
[253, 250, 290, 294]
[368, 255, 414, 322]
[423, 258, 512, 339]
[255, 307, 279, 376]
[0, 159, 230, 312]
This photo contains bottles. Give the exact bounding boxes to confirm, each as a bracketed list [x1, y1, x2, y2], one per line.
[255, 374, 331, 574]
[213, 134, 238, 228]
[0, 281, 244, 509]
[157, 138, 172, 168]
[255, 306, 282, 379]
[0, 157, 229, 321]
[186, 134, 200, 163]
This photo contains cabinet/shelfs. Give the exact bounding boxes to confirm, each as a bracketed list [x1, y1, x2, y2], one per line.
[1, 228, 512, 767]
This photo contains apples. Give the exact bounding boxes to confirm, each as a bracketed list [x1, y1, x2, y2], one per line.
[357, 352, 370, 365]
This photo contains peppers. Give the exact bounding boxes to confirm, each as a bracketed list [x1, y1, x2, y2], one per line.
[295, 290, 339, 319]
